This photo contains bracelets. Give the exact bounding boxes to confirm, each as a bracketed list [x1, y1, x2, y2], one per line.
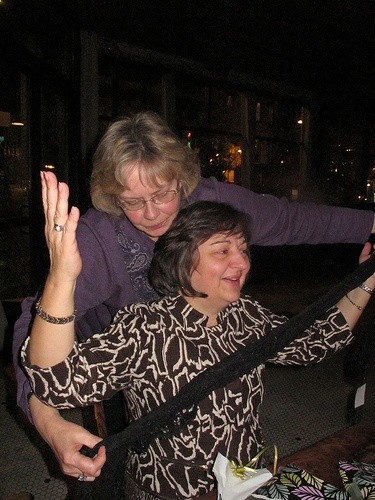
[360, 283, 374, 294]
[346, 294, 365, 311]
[34, 297, 75, 325]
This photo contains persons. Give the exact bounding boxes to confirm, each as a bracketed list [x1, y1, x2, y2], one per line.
[12, 112, 375, 482]
[20, 169, 375, 500]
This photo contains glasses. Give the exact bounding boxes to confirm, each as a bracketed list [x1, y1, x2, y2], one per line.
[115, 179, 180, 212]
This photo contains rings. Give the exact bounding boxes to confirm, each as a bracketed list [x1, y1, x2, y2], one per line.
[54, 224, 64, 231]
[78, 474, 84, 482]
